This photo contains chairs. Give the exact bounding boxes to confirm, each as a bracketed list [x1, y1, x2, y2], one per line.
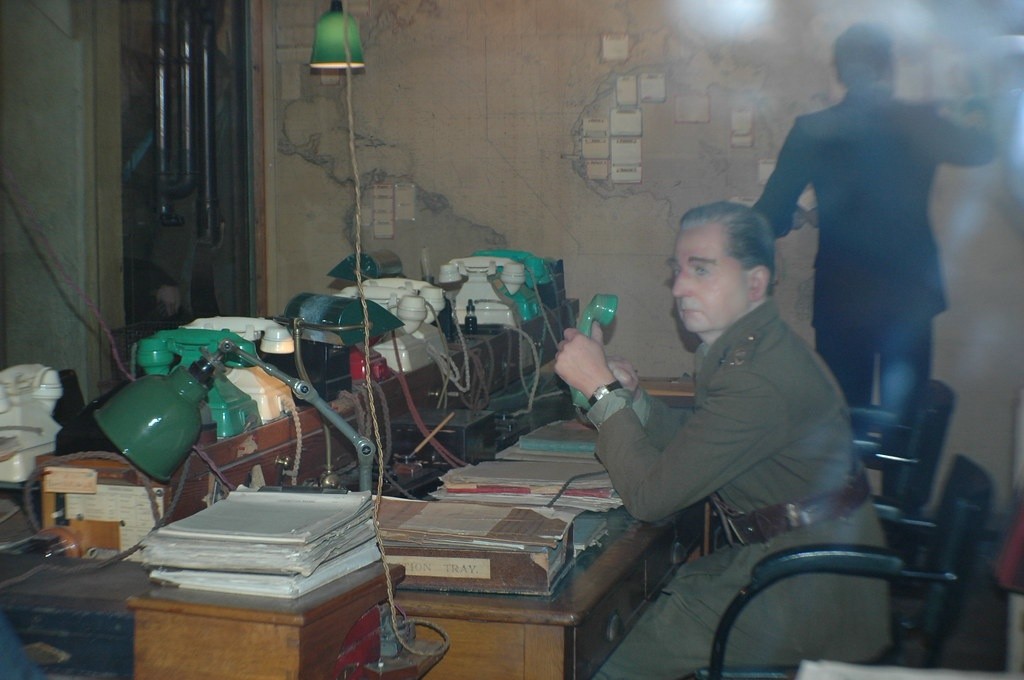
[686, 450, 996, 680]
[847, 376, 958, 517]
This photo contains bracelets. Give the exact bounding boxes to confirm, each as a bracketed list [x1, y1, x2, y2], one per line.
[588, 380, 623, 407]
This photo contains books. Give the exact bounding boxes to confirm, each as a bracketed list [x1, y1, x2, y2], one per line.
[370, 413, 635, 597]
[140, 481, 383, 601]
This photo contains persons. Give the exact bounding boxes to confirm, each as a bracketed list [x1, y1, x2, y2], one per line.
[750, 16, 1004, 501]
[552, 200, 894, 680]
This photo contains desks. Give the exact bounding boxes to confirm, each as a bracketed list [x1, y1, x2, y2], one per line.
[36, 296, 580, 562]
[0, 552, 165, 680]
[386, 378, 726, 680]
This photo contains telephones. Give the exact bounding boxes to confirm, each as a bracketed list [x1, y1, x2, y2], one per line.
[360, 277, 448, 364]
[328, 285, 430, 375]
[566, 293, 619, 411]
[1, 363, 64, 484]
[468, 250, 552, 323]
[180, 315, 295, 426]
[136, 327, 261, 441]
[438, 255, 525, 330]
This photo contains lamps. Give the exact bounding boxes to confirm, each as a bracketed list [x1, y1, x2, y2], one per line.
[309, 0, 366, 72]
[94, 338, 451, 680]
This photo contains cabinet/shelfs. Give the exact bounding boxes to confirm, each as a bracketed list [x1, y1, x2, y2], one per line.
[124, 562, 408, 679]
